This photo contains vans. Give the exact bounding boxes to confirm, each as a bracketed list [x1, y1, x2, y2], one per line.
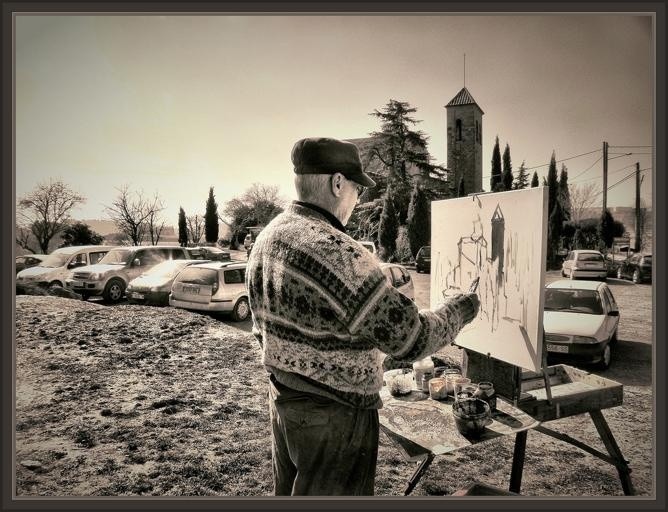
[169, 259, 251, 322]
[16, 246, 122, 298]
[378, 263, 415, 305]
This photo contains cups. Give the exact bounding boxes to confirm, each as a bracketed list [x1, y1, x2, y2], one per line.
[385, 368, 414, 395]
[429, 365, 478, 401]
[451, 398, 497, 442]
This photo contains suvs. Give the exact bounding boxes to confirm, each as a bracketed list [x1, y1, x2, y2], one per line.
[415, 244, 431, 275]
[65, 246, 191, 303]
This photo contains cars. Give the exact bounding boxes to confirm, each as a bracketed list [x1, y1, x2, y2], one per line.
[355, 240, 377, 258]
[125, 259, 212, 306]
[542, 280, 622, 371]
[561, 248, 608, 282]
[617, 251, 652, 285]
[186, 248, 209, 260]
[16, 253, 50, 275]
[193, 247, 231, 261]
[243, 227, 262, 251]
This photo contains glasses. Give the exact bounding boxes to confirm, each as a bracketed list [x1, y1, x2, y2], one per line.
[357, 185, 367, 199]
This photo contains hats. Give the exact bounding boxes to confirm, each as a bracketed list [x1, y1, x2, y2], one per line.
[291, 136, 378, 189]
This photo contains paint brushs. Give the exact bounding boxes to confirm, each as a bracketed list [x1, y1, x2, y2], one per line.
[474, 277, 480, 293]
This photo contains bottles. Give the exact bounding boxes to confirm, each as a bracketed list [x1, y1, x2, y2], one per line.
[476, 383, 498, 414]
[413, 357, 436, 389]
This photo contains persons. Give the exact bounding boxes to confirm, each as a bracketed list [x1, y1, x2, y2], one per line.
[245, 138, 481, 496]
